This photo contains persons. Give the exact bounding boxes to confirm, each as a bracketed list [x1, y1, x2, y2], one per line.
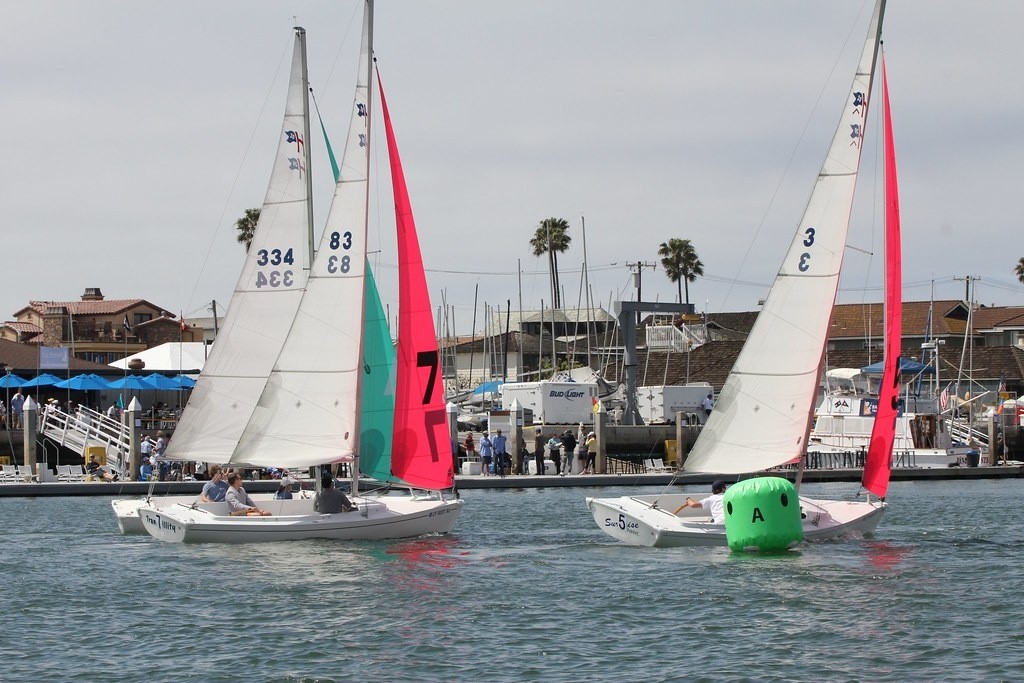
[686, 480, 727, 524]
[98, 327, 116, 341]
[202, 465, 229, 502]
[701, 393, 713, 417]
[224, 473, 272, 516]
[313, 477, 351, 514]
[0, 393, 288, 481]
[451, 425, 597, 475]
[997, 432, 1004, 465]
[964, 389, 970, 400]
[273, 477, 295, 500]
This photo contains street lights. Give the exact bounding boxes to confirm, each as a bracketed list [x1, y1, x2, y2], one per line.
[5, 366, 13, 431]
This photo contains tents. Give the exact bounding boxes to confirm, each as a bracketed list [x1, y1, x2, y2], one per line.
[861, 358, 935, 400]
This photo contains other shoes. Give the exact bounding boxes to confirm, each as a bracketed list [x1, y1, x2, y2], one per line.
[559, 472, 564, 475]
[112, 475, 118, 479]
[579, 471, 585, 475]
[114, 479, 119, 482]
[535, 473, 540, 475]
[567, 472, 571, 475]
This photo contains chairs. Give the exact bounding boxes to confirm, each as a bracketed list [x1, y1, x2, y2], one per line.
[642, 459, 673, 474]
[0, 464, 113, 483]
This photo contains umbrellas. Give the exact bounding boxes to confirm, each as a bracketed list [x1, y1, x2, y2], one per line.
[0, 373, 197, 406]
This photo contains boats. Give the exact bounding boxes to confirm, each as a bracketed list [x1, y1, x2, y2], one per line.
[815, 281, 988, 467]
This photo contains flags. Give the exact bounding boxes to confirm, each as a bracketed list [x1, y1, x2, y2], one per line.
[181, 317, 185, 330]
[123, 315, 131, 331]
[940, 384, 951, 410]
[998, 374, 1006, 392]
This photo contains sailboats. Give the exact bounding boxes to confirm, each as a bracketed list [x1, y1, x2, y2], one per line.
[585, 0, 905, 554]
[137, 2, 465, 547]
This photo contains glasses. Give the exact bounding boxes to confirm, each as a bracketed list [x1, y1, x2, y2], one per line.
[238, 477, 242, 481]
[90, 456, 95, 458]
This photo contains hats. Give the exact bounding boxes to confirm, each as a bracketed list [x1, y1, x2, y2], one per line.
[48, 398, 54, 401]
[143, 457, 150, 461]
[280, 477, 294, 487]
[52, 400, 58, 404]
[713, 480, 725, 488]
[483, 431, 490, 435]
[588, 431, 594, 436]
